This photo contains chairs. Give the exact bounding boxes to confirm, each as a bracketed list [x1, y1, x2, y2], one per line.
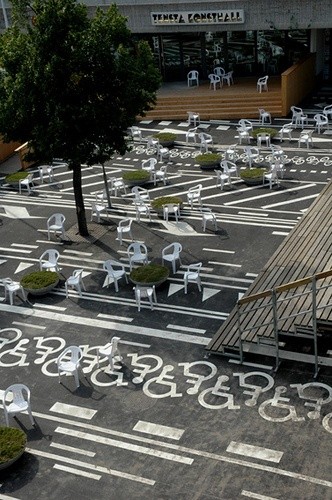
[0, 67, 332, 312]
[2, 336, 122, 427]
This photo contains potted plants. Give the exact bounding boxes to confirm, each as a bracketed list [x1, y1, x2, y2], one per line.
[0, 424, 27, 470]
[239, 167, 268, 185]
[20, 270, 60, 296]
[251, 127, 278, 143]
[194, 153, 223, 168]
[121, 169, 151, 187]
[151, 197, 185, 214]
[151, 133, 177, 147]
[129, 264, 171, 288]
[4, 171, 32, 187]
